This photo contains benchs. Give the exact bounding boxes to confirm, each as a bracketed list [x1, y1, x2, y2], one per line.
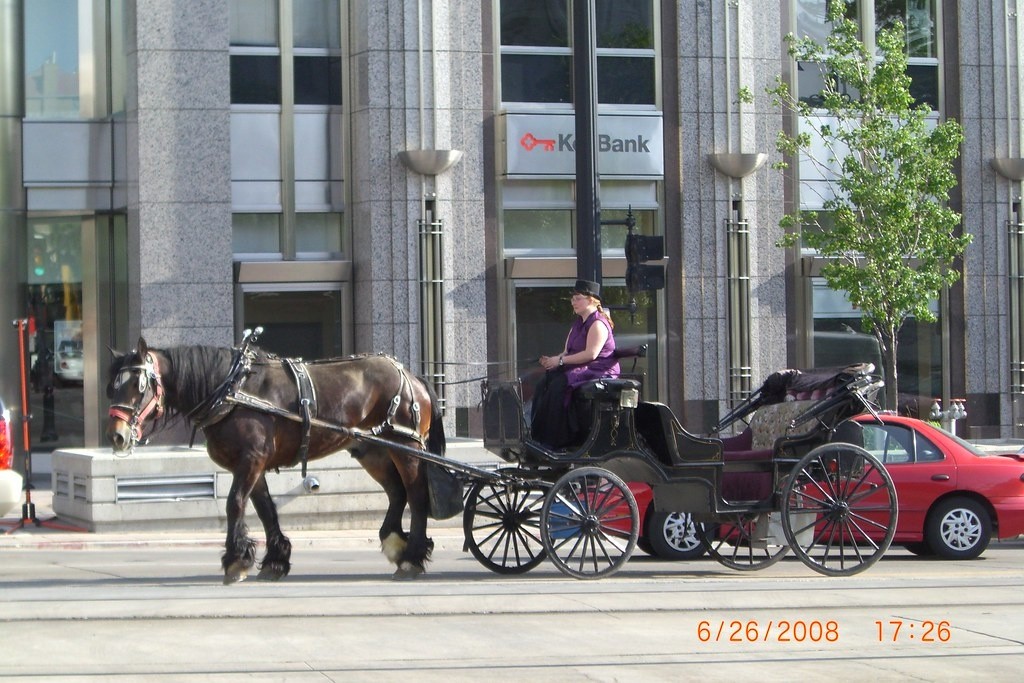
[639, 402, 723, 463]
[720, 363, 876, 501]
[576, 343, 648, 401]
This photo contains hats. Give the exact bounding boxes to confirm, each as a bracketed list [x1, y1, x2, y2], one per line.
[575, 279, 600, 296]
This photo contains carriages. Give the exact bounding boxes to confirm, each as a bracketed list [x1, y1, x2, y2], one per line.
[106, 326, 899, 587]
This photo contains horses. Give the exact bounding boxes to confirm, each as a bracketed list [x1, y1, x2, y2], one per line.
[105, 336, 448, 585]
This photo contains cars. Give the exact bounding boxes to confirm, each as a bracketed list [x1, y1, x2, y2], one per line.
[54, 338, 84, 386]
[575, 409, 1024, 563]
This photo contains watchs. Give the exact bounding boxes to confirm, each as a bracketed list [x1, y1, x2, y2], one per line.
[559, 356, 564, 366]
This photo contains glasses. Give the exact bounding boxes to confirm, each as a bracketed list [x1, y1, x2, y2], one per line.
[571, 297, 589, 305]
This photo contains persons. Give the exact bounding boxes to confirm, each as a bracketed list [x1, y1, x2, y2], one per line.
[539, 279, 620, 445]
